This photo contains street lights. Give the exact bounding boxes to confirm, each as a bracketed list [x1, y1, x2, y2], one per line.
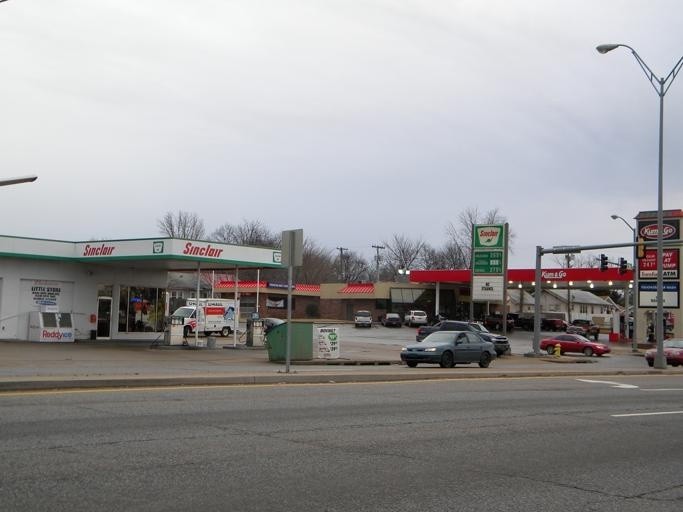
[596, 42, 683, 370]
[609, 215, 639, 352]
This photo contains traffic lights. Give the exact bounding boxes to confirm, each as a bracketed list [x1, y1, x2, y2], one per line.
[601, 254, 608, 272]
[620, 258, 627, 274]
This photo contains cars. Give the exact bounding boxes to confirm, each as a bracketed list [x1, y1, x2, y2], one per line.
[353, 309, 611, 368]
[260, 318, 285, 331]
[645, 337, 683, 367]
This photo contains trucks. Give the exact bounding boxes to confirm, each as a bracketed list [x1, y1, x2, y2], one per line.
[172, 297, 240, 337]
